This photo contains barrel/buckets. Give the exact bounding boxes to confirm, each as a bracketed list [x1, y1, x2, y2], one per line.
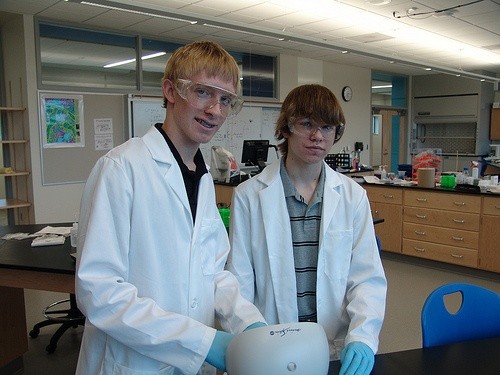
[417, 168, 435, 188]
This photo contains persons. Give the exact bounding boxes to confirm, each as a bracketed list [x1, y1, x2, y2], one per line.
[228, 85, 388, 375]
[74, 41, 269, 375]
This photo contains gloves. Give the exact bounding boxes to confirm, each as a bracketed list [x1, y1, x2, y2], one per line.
[204, 330, 235, 372]
[242, 322, 267, 332]
[339, 341, 375, 375]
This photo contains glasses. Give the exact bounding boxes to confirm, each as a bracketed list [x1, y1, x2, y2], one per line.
[287, 115, 344, 141]
[173, 78, 245, 120]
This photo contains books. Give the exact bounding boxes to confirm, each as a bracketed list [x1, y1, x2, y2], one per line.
[31, 236, 65, 247]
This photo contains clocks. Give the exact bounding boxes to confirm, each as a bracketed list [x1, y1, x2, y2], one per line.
[342, 86, 353, 102]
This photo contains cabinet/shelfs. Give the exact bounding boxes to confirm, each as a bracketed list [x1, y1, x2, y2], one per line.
[364, 186, 500, 273]
[0, 107, 32, 210]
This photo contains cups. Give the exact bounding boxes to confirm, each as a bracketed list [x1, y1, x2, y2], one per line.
[374, 170, 381, 180]
[491, 176, 499, 185]
[397, 171, 406, 179]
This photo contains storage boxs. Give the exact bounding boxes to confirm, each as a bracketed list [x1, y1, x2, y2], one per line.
[325, 153, 350, 170]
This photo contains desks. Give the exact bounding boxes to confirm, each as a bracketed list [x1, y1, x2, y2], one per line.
[327, 335, 500, 375]
[478, 160, 500, 178]
[0, 222, 78, 371]
[214, 174, 254, 209]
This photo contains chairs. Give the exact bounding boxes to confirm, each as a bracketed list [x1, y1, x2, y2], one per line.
[421, 282, 500, 348]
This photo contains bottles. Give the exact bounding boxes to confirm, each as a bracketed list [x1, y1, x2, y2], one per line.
[464, 167, 469, 174]
[71, 219, 79, 248]
[378, 166, 386, 179]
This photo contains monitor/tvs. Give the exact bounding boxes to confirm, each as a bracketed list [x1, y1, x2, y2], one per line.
[241, 139, 269, 166]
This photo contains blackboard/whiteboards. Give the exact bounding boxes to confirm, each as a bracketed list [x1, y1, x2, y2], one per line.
[127, 93, 285, 170]
